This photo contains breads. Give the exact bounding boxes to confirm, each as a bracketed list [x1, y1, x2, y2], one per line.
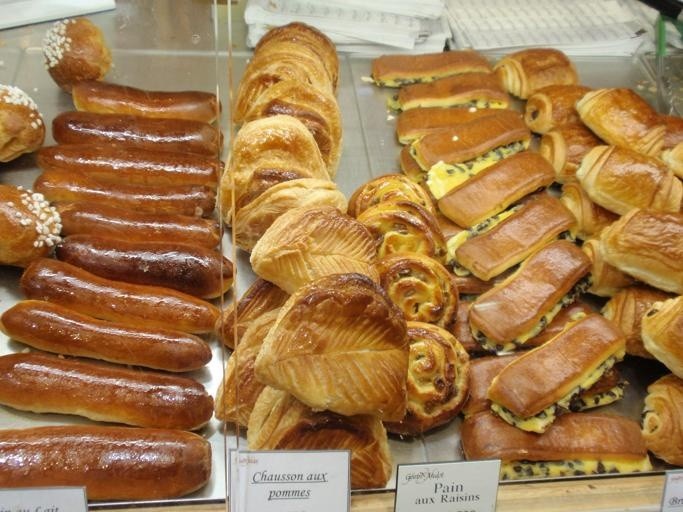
[0, 18, 228, 503]
[217, 16, 682, 483]
